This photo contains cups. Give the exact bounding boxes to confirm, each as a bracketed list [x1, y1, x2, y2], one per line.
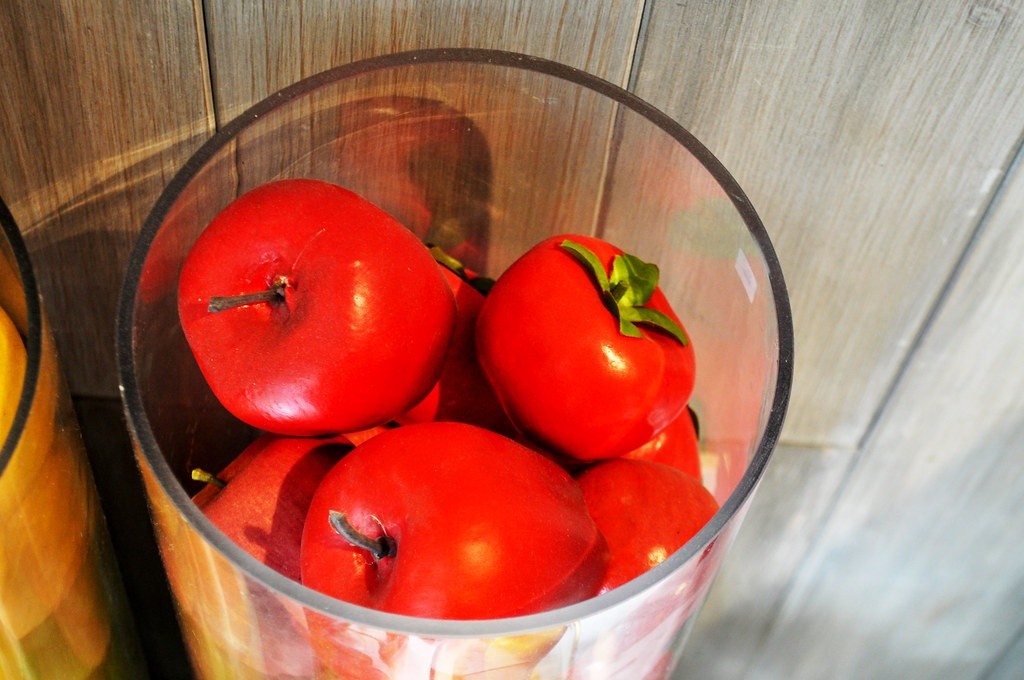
[0, 194, 112, 680]
[114, 44, 796, 680]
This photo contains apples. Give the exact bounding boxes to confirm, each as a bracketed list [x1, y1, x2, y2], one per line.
[176, 178, 720, 680]
